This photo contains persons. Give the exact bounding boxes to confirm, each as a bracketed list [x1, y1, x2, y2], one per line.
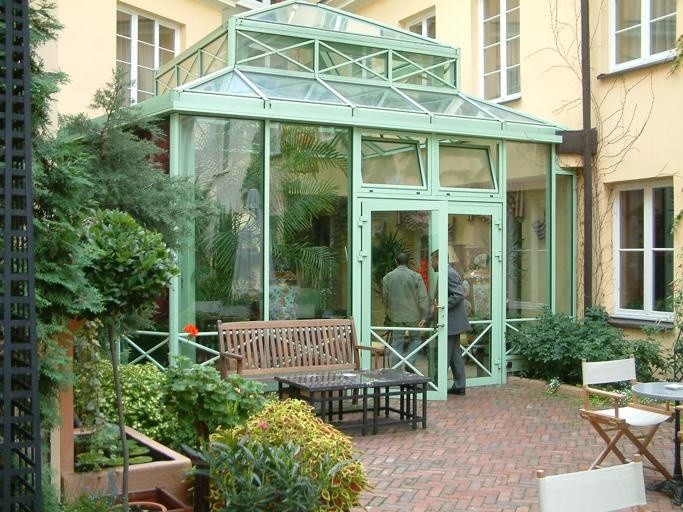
[382, 252, 431, 375]
[417, 249, 473, 395]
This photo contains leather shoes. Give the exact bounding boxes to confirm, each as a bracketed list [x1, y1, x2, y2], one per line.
[448, 384, 464, 395]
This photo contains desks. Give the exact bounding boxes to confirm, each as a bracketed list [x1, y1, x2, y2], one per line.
[631, 382, 683, 481]
[273, 369, 432, 435]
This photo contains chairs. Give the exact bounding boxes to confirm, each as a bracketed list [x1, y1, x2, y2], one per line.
[537, 454, 647, 512]
[579, 353, 675, 481]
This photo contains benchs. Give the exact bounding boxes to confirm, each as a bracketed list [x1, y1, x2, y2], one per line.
[217, 316, 384, 381]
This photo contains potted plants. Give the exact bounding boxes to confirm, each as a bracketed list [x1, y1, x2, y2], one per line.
[53, 207, 168, 511]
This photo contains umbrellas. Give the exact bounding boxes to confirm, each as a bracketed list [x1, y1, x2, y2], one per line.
[230, 187, 278, 323]
